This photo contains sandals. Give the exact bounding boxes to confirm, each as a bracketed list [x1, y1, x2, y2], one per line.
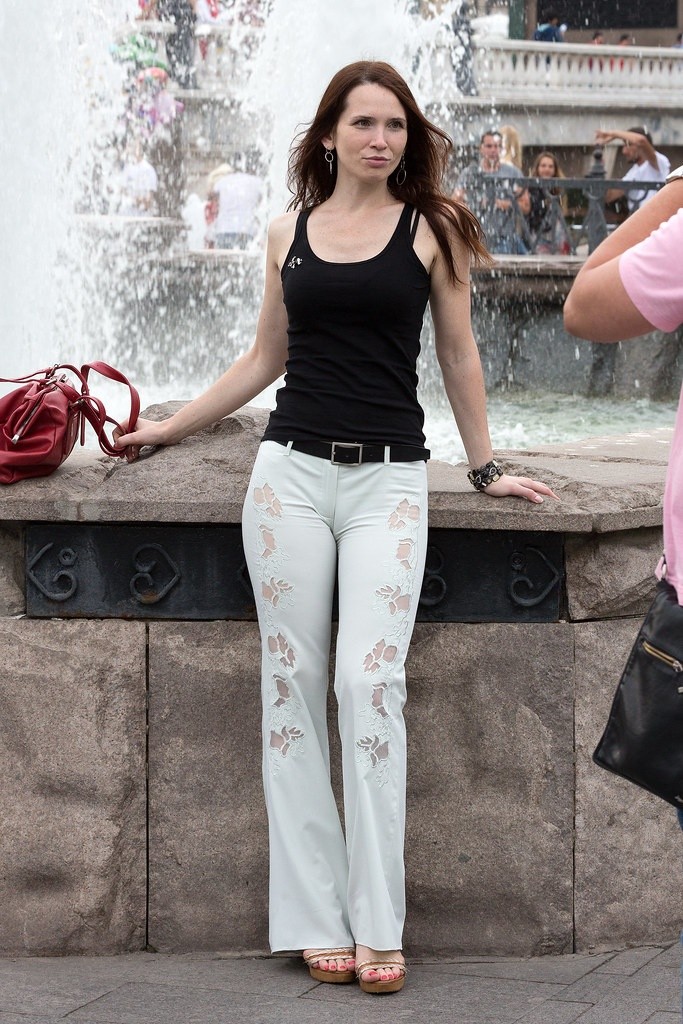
[303, 948, 406, 993]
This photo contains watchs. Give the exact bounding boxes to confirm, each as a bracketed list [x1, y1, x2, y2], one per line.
[664, 165, 683, 184]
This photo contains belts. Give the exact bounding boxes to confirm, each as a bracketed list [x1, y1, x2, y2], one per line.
[262, 432, 431, 465]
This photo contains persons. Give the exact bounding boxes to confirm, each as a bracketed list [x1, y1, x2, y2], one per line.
[110, 57, 560, 996]
[562, 165, 683, 607]
[496, 126, 523, 172]
[450, 131, 531, 255]
[532, 10, 682, 74]
[596, 124, 673, 216]
[526, 152, 570, 254]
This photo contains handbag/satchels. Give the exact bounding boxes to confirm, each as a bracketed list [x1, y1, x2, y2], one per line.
[0, 360, 140, 484]
[590, 587, 683, 808]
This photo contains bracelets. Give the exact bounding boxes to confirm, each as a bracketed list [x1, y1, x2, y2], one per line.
[466, 460, 504, 490]
